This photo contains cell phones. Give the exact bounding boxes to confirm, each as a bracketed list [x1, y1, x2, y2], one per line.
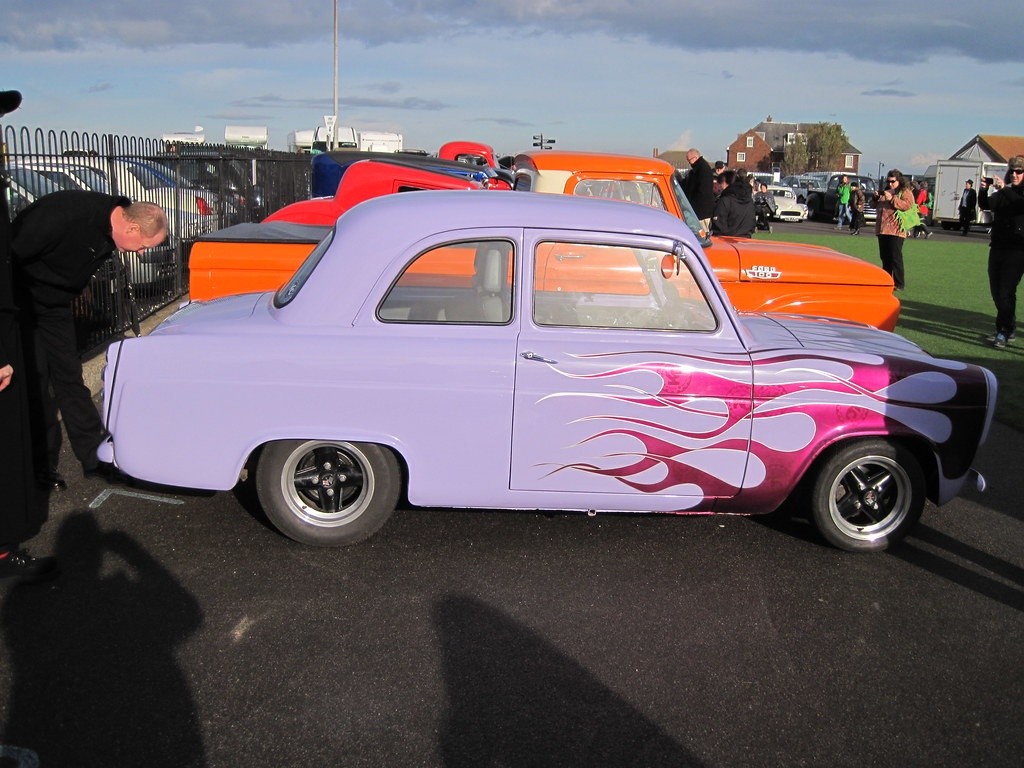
[878, 191, 885, 195]
[984, 178, 994, 184]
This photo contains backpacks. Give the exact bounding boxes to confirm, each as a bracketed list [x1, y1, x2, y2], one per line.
[912, 188, 923, 203]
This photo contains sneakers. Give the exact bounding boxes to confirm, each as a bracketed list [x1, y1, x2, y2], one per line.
[1, 548, 52, 581]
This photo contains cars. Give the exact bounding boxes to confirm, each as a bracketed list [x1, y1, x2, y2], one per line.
[746, 172, 783, 191]
[188, 143, 901, 333]
[4, 156, 263, 318]
[97, 189, 998, 553]
[766, 188, 808, 223]
[783, 176, 828, 204]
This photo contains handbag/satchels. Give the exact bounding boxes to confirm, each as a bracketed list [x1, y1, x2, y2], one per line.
[893, 189, 926, 234]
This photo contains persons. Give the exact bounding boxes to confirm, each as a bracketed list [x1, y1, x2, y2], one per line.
[10, 190, 168, 493]
[834, 174, 865, 235]
[909, 181, 933, 239]
[162, 145, 182, 172]
[0, 90, 59, 582]
[958, 179, 976, 236]
[675, 148, 775, 239]
[869, 169, 914, 291]
[978, 156, 1024, 349]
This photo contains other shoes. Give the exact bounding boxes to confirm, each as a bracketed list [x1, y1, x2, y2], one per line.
[1007, 331, 1015, 342]
[960, 232, 967, 237]
[834, 227, 841, 230]
[925, 231, 933, 239]
[894, 286, 903, 291]
[993, 330, 1007, 348]
[851, 229, 859, 236]
[916, 231, 921, 237]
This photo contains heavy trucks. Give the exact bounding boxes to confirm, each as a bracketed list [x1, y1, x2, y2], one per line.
[287, 130, 314, 154]
[161, 131, 205, 155]
[225, 126, 269, 150]
[310, 127, 403, 155]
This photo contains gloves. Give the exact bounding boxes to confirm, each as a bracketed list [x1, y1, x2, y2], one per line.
[970, 209, 975, 213]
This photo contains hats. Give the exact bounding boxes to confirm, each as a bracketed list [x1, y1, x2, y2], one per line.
[715, 161, 725, 169]
[850, 182, 858, 187]
[1004, 155, 1024, 184]
[965, 179, 974, 185]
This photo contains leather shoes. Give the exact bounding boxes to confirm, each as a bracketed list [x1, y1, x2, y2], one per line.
[84, 463, 119, 480]
[34, 468, 67, 491]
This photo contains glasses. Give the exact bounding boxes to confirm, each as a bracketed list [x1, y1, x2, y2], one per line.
[1009, 169, 1024, 174]
[687, 156, 695, 163]
[886, 179, 897, 184]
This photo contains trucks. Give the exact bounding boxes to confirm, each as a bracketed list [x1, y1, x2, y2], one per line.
[805, 174, 878, 222]
[932, 159, 1008, 229]
